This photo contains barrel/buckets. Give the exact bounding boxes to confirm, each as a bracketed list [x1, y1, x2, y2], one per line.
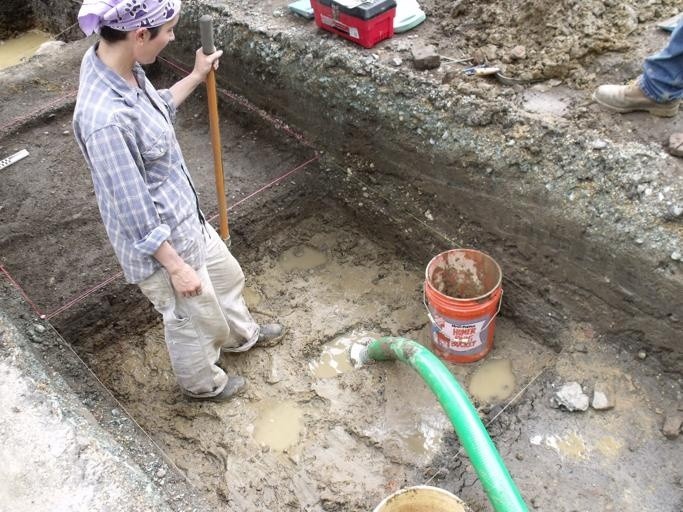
[423, 247, 504, 365]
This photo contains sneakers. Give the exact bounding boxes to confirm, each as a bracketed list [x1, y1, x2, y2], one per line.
[189, 374, 249, 402]
[248, 323, 285, 346]
[592, 78, 680, 118]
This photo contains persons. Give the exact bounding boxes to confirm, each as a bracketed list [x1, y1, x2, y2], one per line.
[72, 0, 286, 402]
[593, 15, 683, 118]
